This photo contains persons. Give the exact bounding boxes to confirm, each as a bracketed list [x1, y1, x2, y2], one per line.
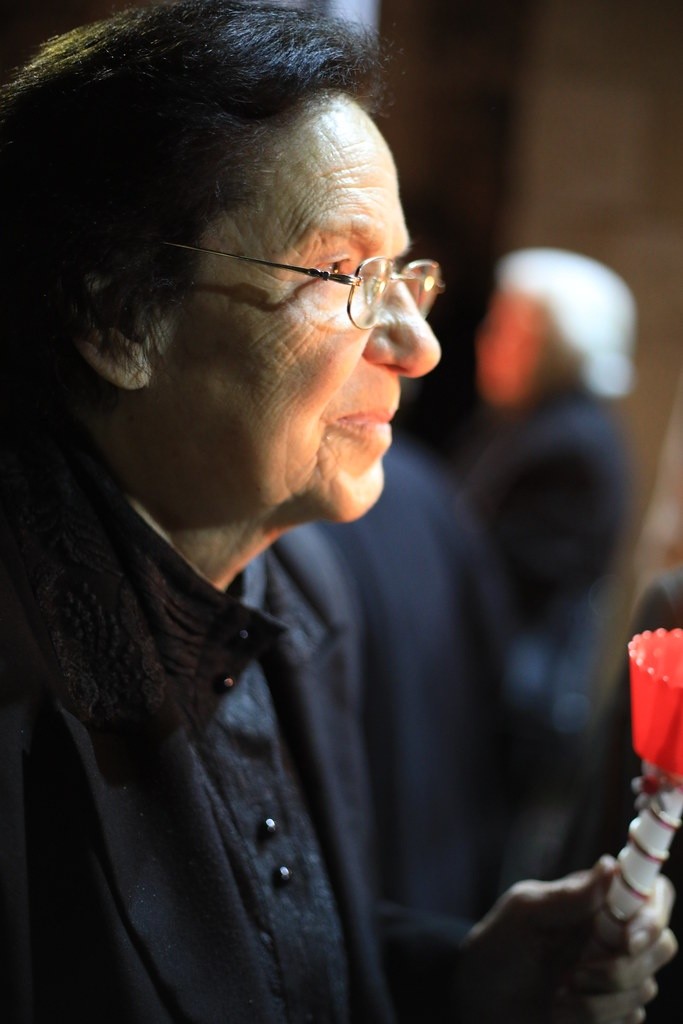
[463, 249, 639, 672]
[0, 0, 677, 1023]
[327, 444, 541, 929]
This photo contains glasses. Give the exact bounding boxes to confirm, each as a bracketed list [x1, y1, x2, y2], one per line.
[157, 237, 443, 329]
